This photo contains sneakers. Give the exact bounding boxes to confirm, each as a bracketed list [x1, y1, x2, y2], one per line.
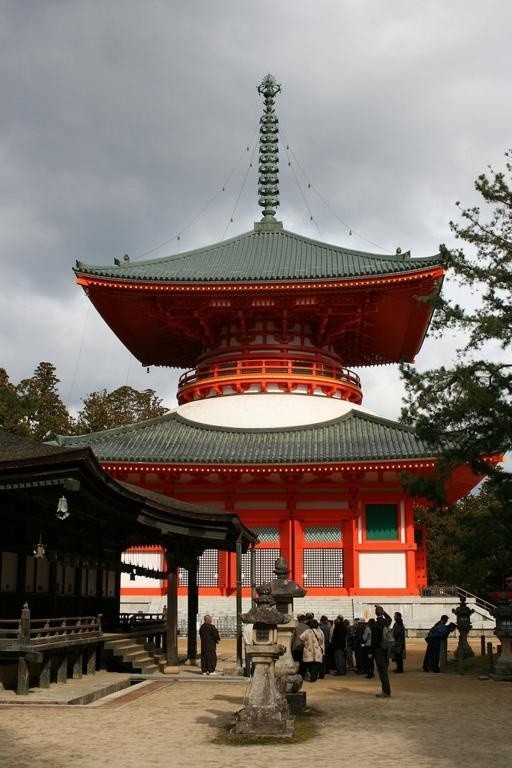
[393, 669, 403, 673]
[209, 673, 217, 675]
[334, 671, 345, 676]
[309, 672, 330, 682]
[376, 692, 390, 697]
[347, 666, 374, 678]
[202, 672, 207, 675]
[423, 666, 440, 672]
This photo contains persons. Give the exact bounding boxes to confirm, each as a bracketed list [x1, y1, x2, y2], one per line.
[199, 615, 221, 675]
[291, 604, 455, 698]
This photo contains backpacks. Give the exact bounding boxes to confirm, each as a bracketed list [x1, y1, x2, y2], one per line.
[381, 626, 395, 649]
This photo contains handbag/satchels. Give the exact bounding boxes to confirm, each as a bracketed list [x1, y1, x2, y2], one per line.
[321, 648, 323, 654]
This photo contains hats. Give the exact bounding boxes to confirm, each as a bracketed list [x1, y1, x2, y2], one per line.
[358, 618, 365, 622]
